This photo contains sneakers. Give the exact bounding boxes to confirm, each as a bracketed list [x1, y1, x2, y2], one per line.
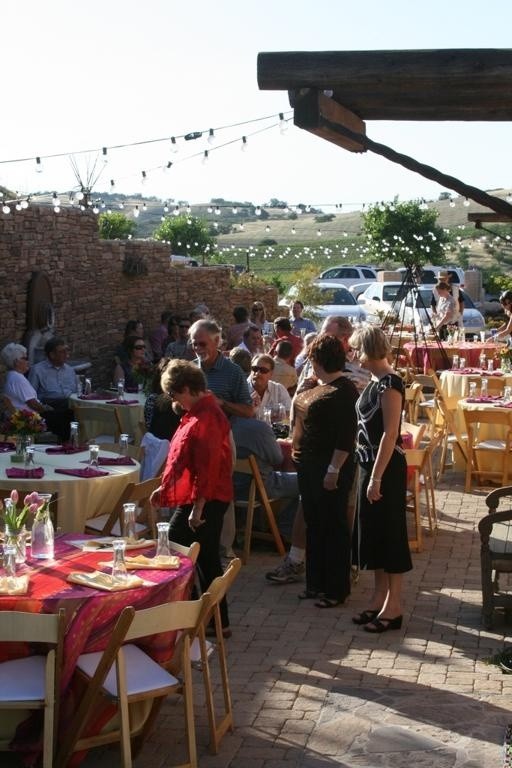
[265, 557, 306, 583]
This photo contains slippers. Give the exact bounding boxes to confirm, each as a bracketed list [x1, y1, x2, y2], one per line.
[363, 615, 402, 633]
[353, 608, 379, 624]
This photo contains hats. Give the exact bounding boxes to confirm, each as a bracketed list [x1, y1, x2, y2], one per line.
[434, 271, 453, 279]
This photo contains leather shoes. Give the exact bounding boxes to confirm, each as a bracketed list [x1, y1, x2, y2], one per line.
[205, 631, 231, 639]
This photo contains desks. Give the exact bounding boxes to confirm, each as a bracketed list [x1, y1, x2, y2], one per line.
[1, 536, 193, 762]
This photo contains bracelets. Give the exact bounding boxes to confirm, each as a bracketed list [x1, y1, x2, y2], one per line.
[369, 477, 381, 482]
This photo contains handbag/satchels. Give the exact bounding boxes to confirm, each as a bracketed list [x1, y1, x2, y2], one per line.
[272, 422, 289, 439]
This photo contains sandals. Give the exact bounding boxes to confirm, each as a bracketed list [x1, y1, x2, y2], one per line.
[315, 594, 347, 609]
[298, 591, 325, 599]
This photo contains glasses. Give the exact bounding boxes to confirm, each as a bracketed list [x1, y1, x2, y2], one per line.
[188, 341, 208, 347]
[251, 365, 271, 374]
[132, 344, 145, 349]
[167, 392, 175, 398]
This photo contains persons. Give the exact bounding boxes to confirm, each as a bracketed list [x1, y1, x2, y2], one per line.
[430, 270, 464, 315]
[430, 282, 465, 342]
[144, 358, 198, 477]
[149, 360, 236, 637]
[292, 335, 360, 608]
[113, 337, 154, 394]
[264, 316, 371, 588]
[251, 302, 276, 337]
[269, 341, 299, 390]
[114, 319, 145, 368]
[248, 356, 291, 425]
[226, 307, 256, 350]
[27, 338, 80, 442]
[486, 288, 512, 342]
[151, 305, 211, 362]
[189, 319, 256, 575]
[288, 302, 317, 334]
[237, 326, 268, 357]
[295, 332, 317, 377]
[2, 343, 72, 441]
[266, 317, 303, 357]
[230, 414, 301, 503]
[348, 326, 406, 633]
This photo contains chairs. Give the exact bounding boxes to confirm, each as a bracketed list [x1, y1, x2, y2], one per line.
[133, 557, 242, 755]
[57, 593, 214, 765]
[478, 483, 512, 631]
[0, 332, 511, 575]
[3, 610, 63, 766]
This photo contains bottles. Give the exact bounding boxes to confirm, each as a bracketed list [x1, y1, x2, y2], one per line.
[468, 378, 511, 404]
[83, 377, 143, 403]
[68, 421, 80, 448]
[1, 542, 21, 589]
[23, 445, 35, 473]
[29, 493, 55, 560]
[123, 502, 138, 542]
[453, 328, 485, 343]
[451, 352, 494, 372]
[153, 521, 172, 564]
[87, 443, 99, 471]
[262, 403, 288, 427]
[110, 539, 128, 585]
[117, 433, 130, 463]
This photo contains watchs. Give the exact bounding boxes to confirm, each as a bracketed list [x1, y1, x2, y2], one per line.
[327, 465, 339, 474]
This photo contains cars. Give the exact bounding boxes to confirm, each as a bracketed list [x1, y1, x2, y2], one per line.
[279, 266, 496, 339]
[172, 253, 205, 266]
